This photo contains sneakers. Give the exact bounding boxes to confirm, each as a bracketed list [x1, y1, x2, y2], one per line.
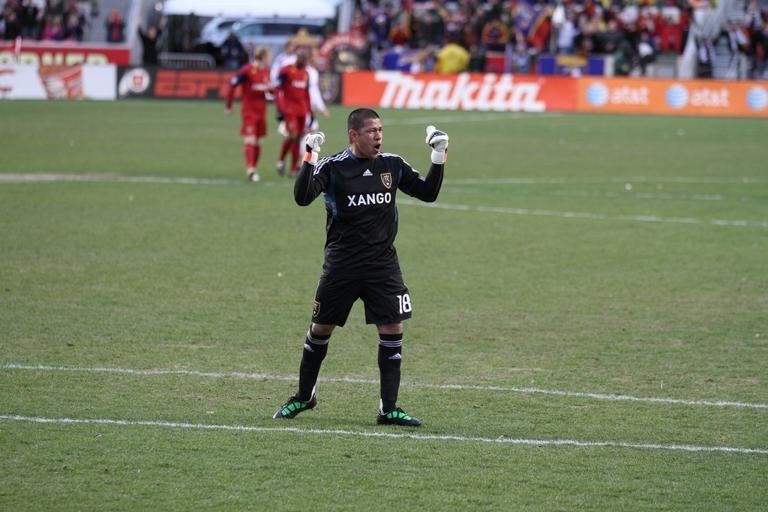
[276, 161, 285, 178]
[377, 406, 421, 427]
[247, 169, 260, 181]
[272, 390, 317, 420]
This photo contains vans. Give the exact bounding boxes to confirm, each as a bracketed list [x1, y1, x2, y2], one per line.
[202, 15, 332, 62]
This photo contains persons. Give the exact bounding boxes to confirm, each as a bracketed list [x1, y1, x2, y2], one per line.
[225, 41, 327, 182]
[274, 108, 448, 425]
[350, 0, 768, 83]
[103, 12, 126, 44]
[0, 0, 92, 44]
[136, 23, 164, 65]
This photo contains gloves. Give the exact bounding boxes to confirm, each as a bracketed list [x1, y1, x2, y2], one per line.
[303, 131, 326, 166]
[425, 125, 449, 165]
